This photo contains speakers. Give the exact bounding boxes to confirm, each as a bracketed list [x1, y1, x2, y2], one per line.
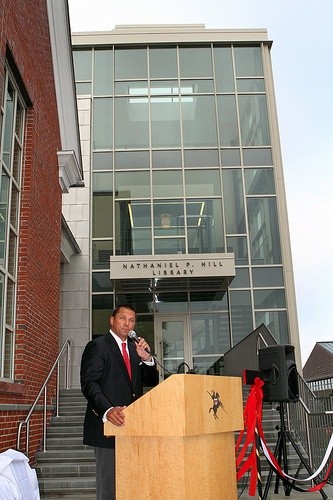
[259, 344, 299, 403]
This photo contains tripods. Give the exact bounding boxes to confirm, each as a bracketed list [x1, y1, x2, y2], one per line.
[261, 403, 327, 500]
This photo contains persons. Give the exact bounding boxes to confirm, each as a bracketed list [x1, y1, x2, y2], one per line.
[80, 306, 159, 500]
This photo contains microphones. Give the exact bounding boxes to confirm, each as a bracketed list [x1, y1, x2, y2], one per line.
[128, 330, 150, 355]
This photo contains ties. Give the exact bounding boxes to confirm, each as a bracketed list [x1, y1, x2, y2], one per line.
[121, 343, 131, 381]
